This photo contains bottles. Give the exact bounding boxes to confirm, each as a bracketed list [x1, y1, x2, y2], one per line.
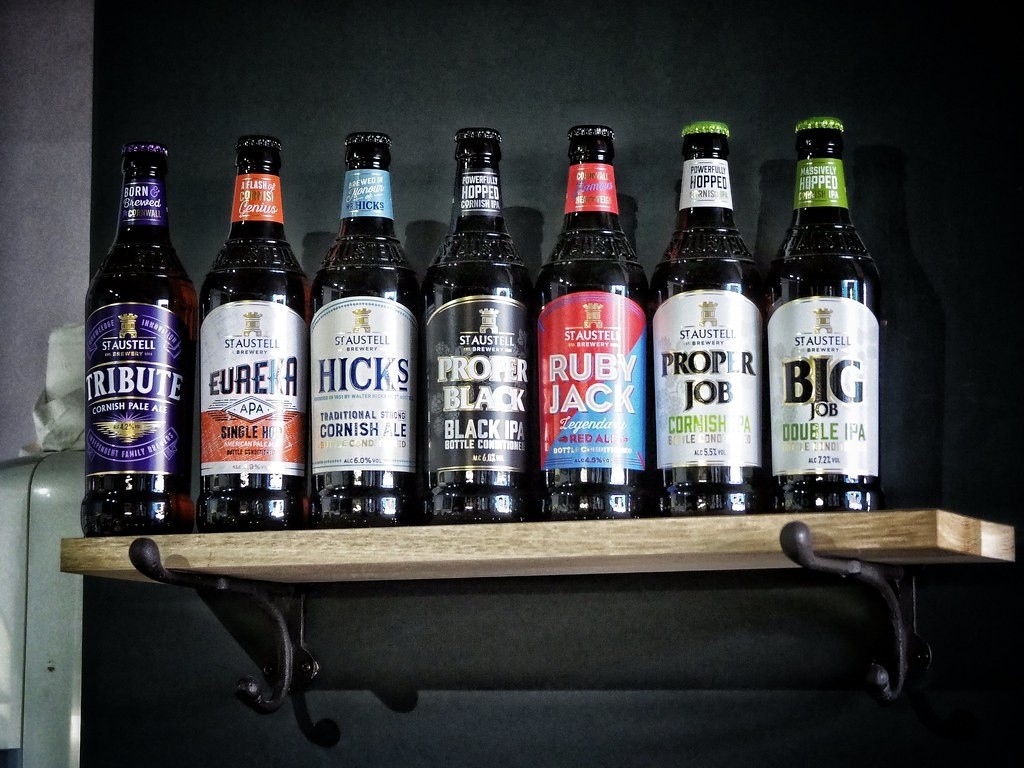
[765, 119, 887, 513]
[649, 122, 766, 516]
[80, 143, 196, 534]
[535, 126, 648, 518]
[195, 137, 307, 532]
[419, 126, 535, 521]
[306, 131, 419, 528]
